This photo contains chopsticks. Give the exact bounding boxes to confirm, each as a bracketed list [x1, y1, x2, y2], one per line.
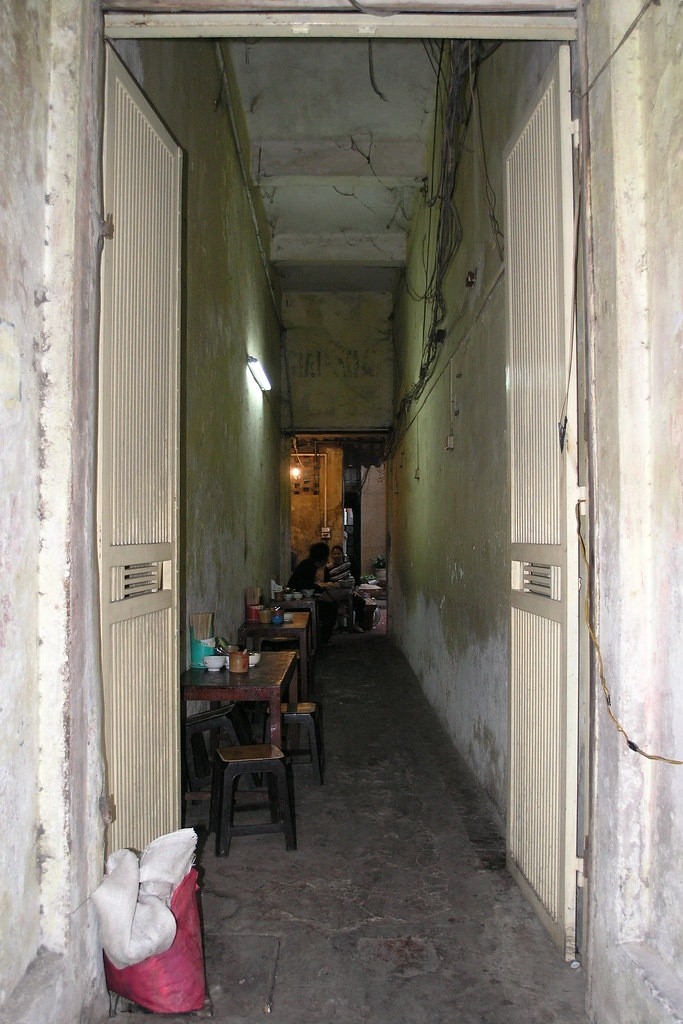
[246, 585, 262, 604]
[189, 612, 212, 640]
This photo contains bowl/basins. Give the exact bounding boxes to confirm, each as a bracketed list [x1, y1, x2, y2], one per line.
[248, 653, 261, 666]
[271, 615, 284, 623]
[202, 656, 226, 671]
[272, 610, 284, 616]
[223, 656, 229, 669]
[284, 612, 293, 621]
[283, 587, 315, 601]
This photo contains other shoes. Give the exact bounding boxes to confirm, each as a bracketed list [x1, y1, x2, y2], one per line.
[354, 624, 364, 632]
[333, 620, 337, 631]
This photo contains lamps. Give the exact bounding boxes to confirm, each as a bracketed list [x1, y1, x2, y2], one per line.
[248, 355, 272, 391]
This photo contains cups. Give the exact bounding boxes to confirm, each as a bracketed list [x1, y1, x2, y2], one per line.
[229, 651, 249, 673]
[259, 610, 272, 623]
[274, 592, 284, 601]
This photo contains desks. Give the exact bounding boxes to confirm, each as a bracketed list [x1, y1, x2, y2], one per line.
[318, 582, 354, 634]
[267, 596, 318, 649]
[181, 651, 300, 823]
[238, 611, 310, 702]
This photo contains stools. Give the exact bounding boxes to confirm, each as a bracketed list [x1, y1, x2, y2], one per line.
[209, 744, 298, 856]
[186, 704, 257, 805]
[261, 700, 324, 787]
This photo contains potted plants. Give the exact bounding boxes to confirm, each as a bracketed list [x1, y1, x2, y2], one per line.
[369, 554, 387, 578]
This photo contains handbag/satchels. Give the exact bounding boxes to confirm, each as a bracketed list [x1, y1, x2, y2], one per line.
[102, 867, 205, 1014]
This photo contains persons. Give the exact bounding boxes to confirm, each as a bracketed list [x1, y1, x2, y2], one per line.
[286, 542, 340, 649]
[323, 546, 365, 634]
